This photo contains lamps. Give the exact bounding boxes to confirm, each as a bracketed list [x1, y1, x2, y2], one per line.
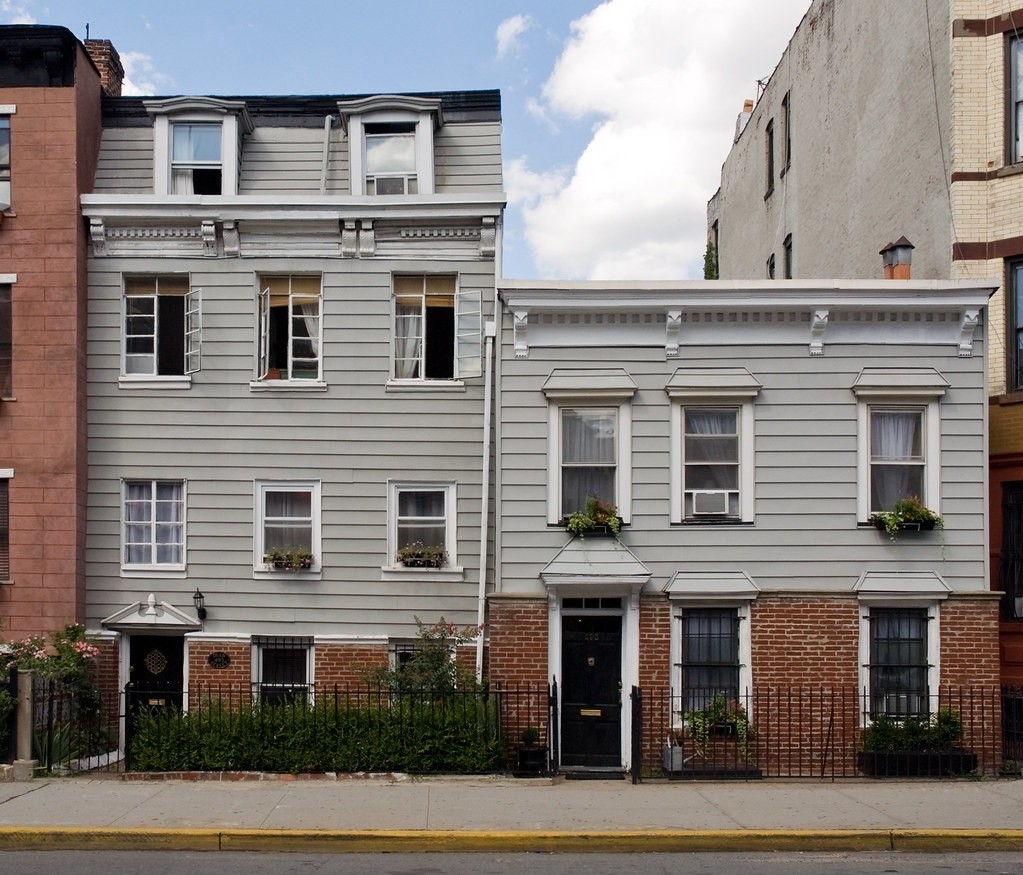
[193, 588, 207, 620]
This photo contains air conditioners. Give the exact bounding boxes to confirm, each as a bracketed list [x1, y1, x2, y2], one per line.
[692, 491, 728, 515]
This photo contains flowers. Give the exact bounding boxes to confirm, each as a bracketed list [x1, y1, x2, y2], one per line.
[393, 539, 450, 569]
[264, 545, 314, 574]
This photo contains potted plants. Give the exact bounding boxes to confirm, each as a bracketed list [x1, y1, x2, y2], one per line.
[856, 704, 979, 779]
[518, 728, 546, 769]
[680, 692, 756, 761]
[870, 492, 946, 563]
[562, 496, 623, 545]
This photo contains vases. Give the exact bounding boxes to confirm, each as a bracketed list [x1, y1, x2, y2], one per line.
[403, 553, 442, 568]
[271, 554, 311, 569]
